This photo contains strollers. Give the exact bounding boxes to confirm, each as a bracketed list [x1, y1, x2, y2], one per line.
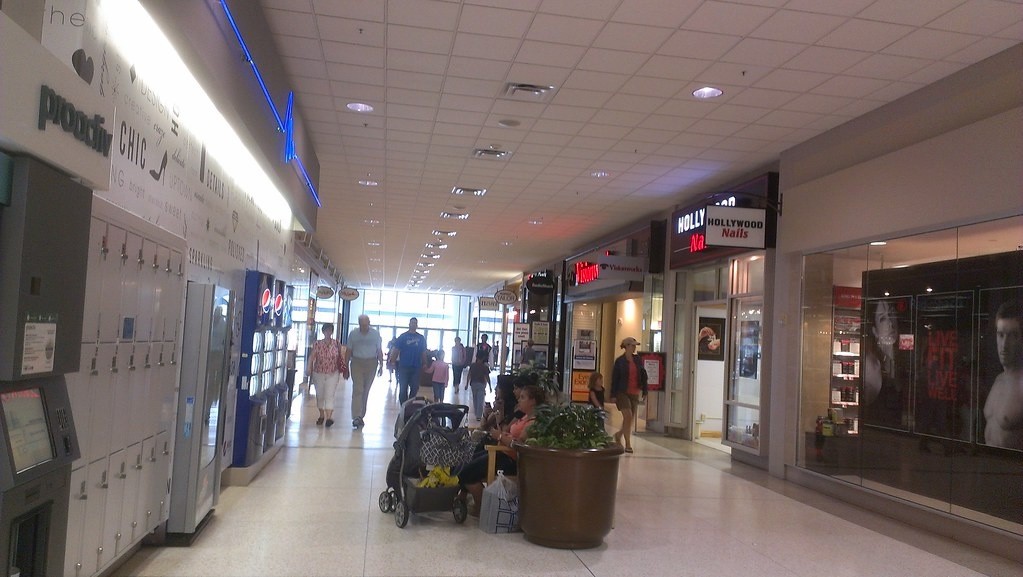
[377, 395, 471, 530]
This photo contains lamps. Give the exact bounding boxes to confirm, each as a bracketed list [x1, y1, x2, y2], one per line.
[607, 250, 615, 256]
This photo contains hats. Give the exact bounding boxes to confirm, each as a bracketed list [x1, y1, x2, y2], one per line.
[622, 337, 640, 347]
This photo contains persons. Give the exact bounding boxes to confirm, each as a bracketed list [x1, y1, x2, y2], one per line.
[863, 300, 907, 429]
[983, 301, 1023, 452]
[587, 372, 605, 430]
[611, 337, 649, 453]
[303, 315, 544, 517]
[700, 326, 720, 354]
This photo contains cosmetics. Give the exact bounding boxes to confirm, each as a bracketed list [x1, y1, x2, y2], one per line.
[833, 338, 861, 434]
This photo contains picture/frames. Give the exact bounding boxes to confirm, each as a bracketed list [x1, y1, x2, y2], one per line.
[698, 316, 726, 361]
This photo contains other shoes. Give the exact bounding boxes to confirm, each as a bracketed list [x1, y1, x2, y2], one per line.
[357, 423, 363, 430]
[624, 447, 633, 453]
[476, 418, 480, 421]
[315, 418, 324, 425]
[613, 432, 620, 445]
[352, 419, 359, 426]
[455, 389, 459, 394]
[325, 419, 333, 427]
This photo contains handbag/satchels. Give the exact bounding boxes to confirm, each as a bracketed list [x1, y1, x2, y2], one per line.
[479, 470, 519, 534]
[336, 340, 347, 372]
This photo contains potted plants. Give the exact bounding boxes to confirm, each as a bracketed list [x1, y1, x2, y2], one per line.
[512, 400, 625, 550]
[419, 349, 438, 387]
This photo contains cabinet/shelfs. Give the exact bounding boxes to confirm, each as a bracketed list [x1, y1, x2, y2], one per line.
[828, 283, 862, 419]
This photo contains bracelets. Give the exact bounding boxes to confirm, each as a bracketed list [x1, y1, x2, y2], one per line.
[497, 433, 505, 441]
